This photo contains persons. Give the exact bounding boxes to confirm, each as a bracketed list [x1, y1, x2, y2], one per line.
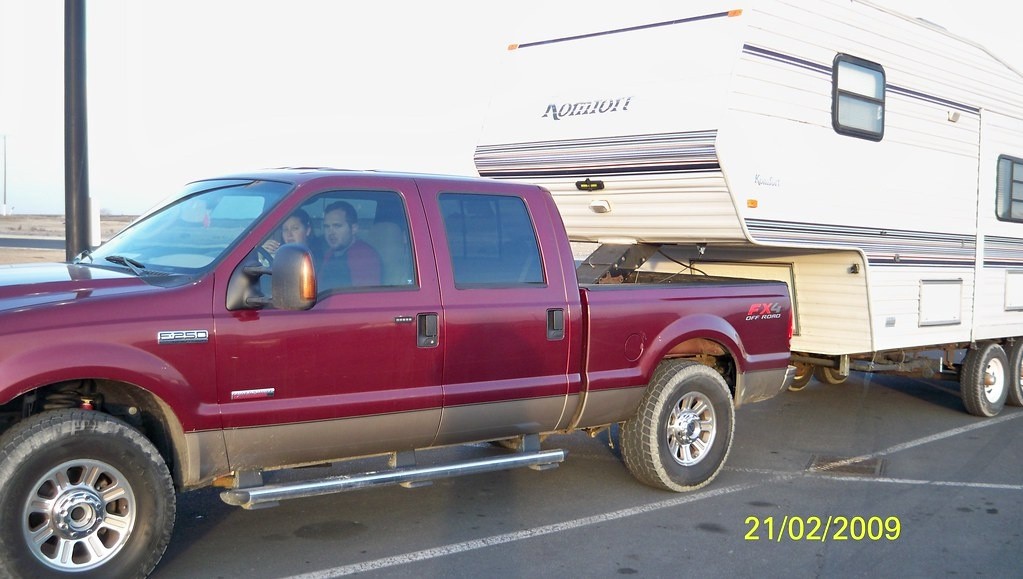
[257, 201, 383, 288]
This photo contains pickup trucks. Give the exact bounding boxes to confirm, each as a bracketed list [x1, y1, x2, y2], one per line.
[0, 169, 793, 579]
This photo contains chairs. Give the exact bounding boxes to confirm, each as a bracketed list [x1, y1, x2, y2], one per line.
[367, 222, 411, 286]
